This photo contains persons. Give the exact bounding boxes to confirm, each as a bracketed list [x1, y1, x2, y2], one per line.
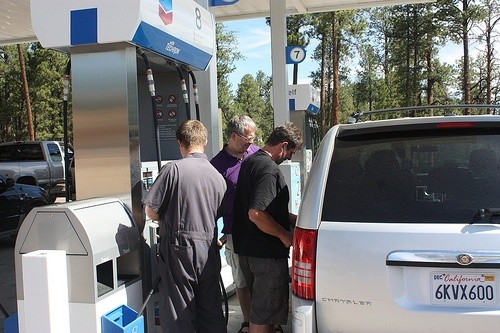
[209, 116, 283, 333]
[231, 123, 304, 333]
[144, 119, 227, 333]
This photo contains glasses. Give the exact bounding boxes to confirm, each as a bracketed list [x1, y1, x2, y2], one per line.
[235, 132, 256, 141]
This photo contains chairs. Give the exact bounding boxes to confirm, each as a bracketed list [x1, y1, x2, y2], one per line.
[367, 158, 416, 206]
[457, 150, 500, 205]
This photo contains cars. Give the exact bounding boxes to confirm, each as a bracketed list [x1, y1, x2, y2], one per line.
[0, 183, 53, 245]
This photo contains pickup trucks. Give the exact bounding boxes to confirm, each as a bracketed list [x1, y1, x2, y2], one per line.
[0, 141, 74, 186]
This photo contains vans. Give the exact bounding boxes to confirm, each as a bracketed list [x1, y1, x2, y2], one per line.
[291, 104, 500, 333]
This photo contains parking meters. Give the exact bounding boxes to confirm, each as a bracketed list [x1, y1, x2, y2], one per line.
[141, 162, 154, 191]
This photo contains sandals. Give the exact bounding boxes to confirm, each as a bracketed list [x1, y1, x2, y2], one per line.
[273, 323, 283, 333]
[238, 321, 249, 333]
